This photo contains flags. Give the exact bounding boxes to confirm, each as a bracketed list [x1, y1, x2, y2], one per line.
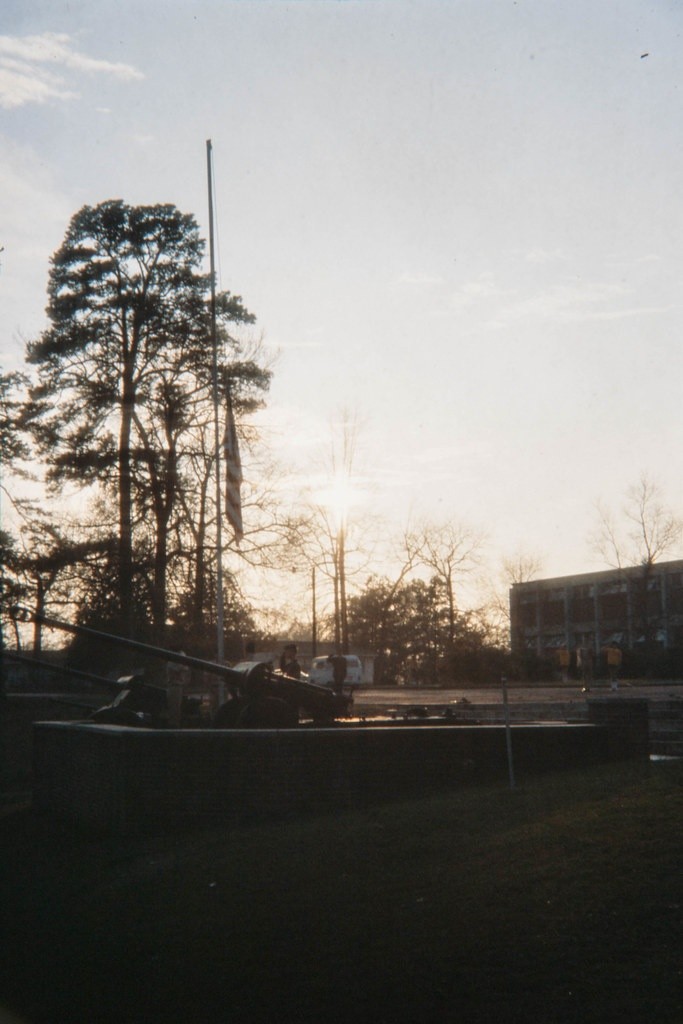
[224, 369, 243, 547]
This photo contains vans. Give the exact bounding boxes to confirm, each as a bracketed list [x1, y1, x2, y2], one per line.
[308, 655, 362, 690]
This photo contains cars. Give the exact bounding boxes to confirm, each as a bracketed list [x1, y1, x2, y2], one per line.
[273, 669, 309, 681]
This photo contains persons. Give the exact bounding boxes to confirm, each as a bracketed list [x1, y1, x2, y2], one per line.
[580, 649, 594, 692]
[280, 644, 301, 679]
[601, 641, 621, 691]
[328, 647, 347, 693]
